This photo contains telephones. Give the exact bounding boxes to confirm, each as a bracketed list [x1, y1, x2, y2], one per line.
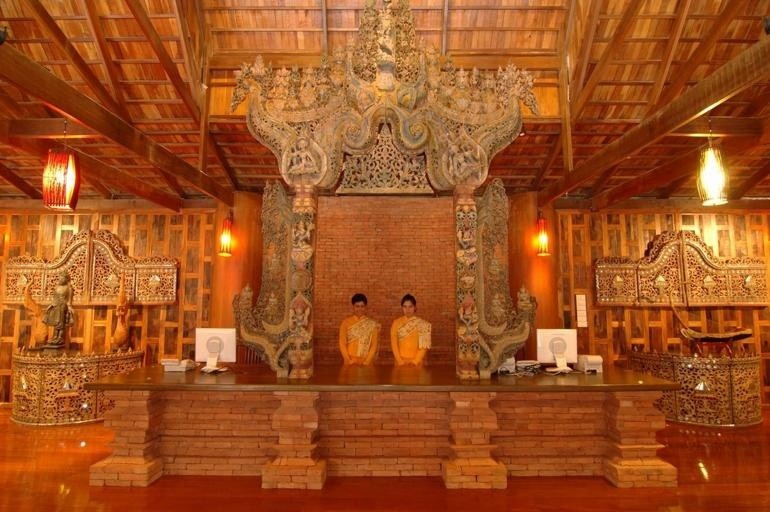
[180, 359, 195, 368]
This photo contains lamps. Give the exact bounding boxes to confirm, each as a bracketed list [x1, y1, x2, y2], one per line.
[41, 116, 79, 213]
[695, 120, 729, 207]
[536, 211, 551, 256]
[217, 208, 232, 256]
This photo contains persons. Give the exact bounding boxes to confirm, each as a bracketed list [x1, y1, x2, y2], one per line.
[390, 293, 433, 367]
[339, 294, 382, 365]
[41, 268, 75, 346]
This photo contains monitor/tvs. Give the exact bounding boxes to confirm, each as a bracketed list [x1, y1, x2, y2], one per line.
[535, 327, 579, 374]
[195, 327, 237, 373]
